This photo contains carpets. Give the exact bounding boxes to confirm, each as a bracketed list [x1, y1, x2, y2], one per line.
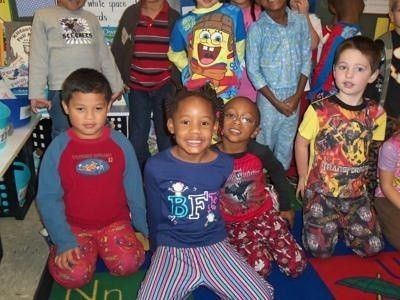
[32, 208, 400, 300]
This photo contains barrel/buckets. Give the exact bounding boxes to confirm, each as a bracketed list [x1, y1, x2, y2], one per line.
[0, 87, 31, 128]
[0, 102, 12, 150]
[0, 162, 31, 212]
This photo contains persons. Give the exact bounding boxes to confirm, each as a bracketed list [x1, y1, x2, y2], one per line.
[373, 112, 400, 209]
[211, 94, 308, 280]
[29, 0, 125, 138]
[136, 85, 276, 300]
[33, 66, 151, 290]
[111, 1, 400, 184]
[295, 33, 388, 259]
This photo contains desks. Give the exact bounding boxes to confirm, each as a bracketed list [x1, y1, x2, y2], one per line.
[1, 87, 132, 152]
[0, 107, 46, 221]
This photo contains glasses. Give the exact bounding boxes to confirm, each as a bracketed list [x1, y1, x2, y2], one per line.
[224, 111, 254, 125]
